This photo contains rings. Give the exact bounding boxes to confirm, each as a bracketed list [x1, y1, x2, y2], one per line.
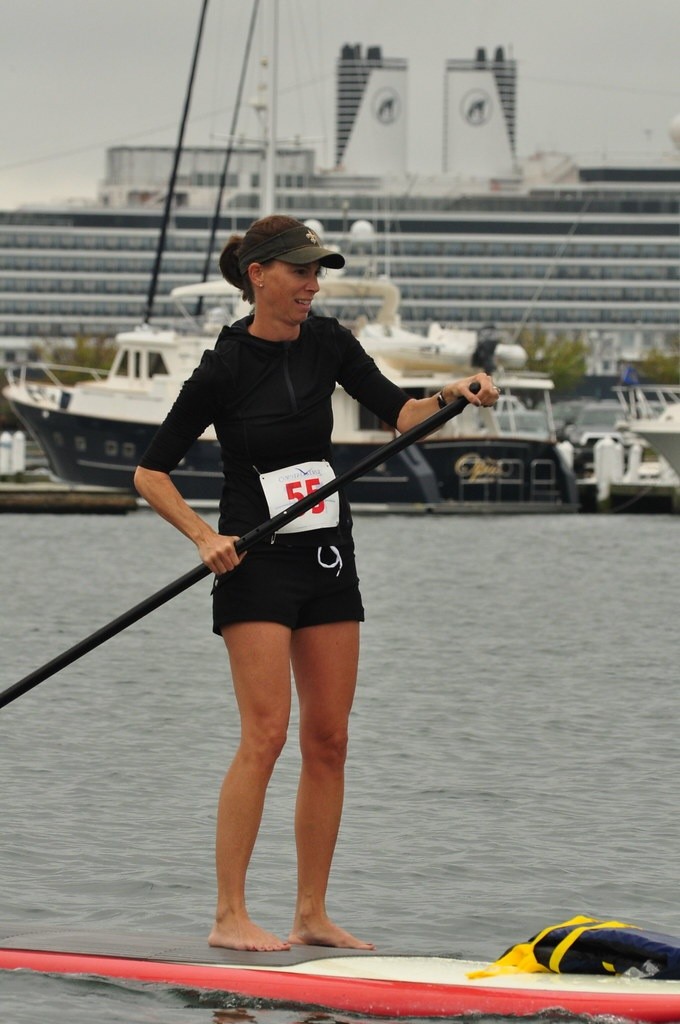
[493, 386, 501, 392]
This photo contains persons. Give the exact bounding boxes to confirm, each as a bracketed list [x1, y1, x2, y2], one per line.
[133, 216, 499, 952]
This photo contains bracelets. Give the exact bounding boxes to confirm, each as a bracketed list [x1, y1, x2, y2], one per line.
[437, 391, 447, 409]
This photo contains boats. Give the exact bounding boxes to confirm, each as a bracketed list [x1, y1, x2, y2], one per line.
[577, 432, 680, 510]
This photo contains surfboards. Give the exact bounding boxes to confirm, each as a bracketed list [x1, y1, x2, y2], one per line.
[0, 922, 680, 1024]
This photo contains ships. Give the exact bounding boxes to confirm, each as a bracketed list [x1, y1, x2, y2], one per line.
[0, 40, 680, 399]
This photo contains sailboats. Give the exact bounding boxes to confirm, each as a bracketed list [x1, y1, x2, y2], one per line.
[0, 0, 570, 514]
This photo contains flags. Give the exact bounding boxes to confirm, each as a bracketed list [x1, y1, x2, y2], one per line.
[622, 367, 640, 386]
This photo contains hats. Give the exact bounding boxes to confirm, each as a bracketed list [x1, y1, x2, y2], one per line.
[239, 226, 345, 276]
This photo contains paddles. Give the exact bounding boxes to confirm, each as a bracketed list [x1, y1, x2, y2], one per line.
[0, 377, 487, 711]
